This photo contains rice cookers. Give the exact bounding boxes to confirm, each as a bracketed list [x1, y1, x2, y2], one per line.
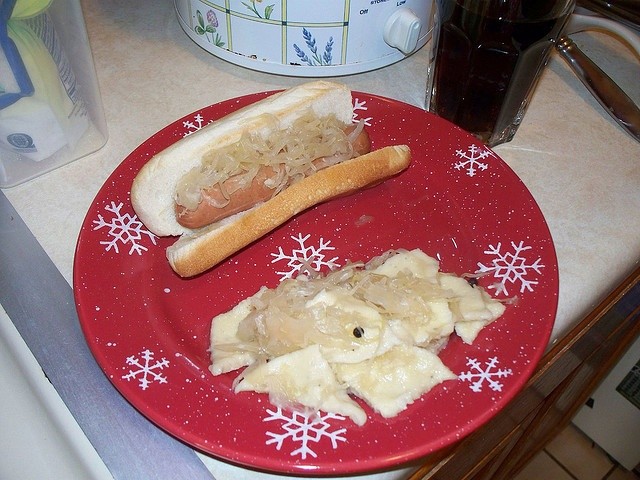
[173, 2, 436, 78]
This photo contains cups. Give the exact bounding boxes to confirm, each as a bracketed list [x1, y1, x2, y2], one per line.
[429, 1, 575, 149]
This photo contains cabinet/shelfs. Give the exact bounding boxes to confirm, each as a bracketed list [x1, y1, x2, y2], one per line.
[410, 261, 640, 480]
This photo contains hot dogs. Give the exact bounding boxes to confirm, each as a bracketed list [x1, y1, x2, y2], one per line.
[131, 77, 413, 278]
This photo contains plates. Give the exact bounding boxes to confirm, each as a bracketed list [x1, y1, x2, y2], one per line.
[73, 87, 560, 475]
